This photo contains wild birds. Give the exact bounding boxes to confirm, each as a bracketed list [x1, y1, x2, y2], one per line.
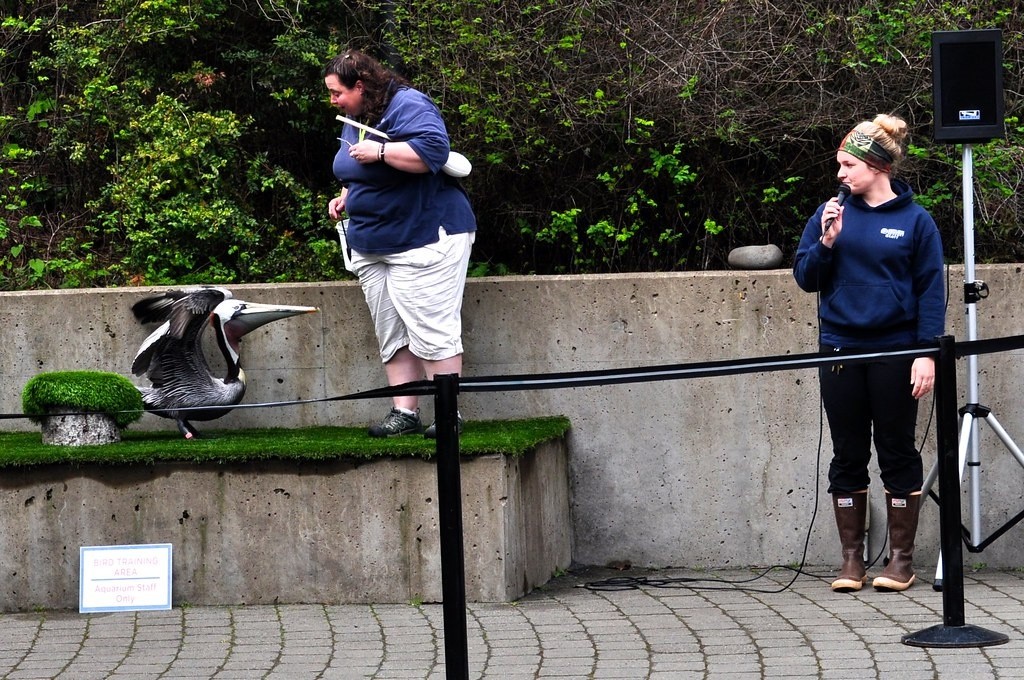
[131, 286, 323, 439]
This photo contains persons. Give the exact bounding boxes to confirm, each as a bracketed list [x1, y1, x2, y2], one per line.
[321, 49, 478, 441]
[793, 114, 946, 591]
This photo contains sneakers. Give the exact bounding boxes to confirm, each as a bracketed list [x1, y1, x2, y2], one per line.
[368, 405, 423, 439]
[424, 409, 464, 439]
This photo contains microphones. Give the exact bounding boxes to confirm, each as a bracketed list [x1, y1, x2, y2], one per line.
[825, 183, 851, 231]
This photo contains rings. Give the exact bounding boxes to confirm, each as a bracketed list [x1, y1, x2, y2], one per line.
[922, 382, 928, 387]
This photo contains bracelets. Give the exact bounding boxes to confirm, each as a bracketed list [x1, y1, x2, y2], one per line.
[377, 142, 385, 162]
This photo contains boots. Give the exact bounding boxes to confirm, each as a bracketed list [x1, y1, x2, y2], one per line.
[873, 488, 922, 591]
[832, 488, 869, 591]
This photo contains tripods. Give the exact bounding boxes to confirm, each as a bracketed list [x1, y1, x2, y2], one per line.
[883, 142, 1024, 591]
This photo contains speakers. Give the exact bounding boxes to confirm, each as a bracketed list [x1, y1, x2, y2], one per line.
[932, 28, 1005, 145]
